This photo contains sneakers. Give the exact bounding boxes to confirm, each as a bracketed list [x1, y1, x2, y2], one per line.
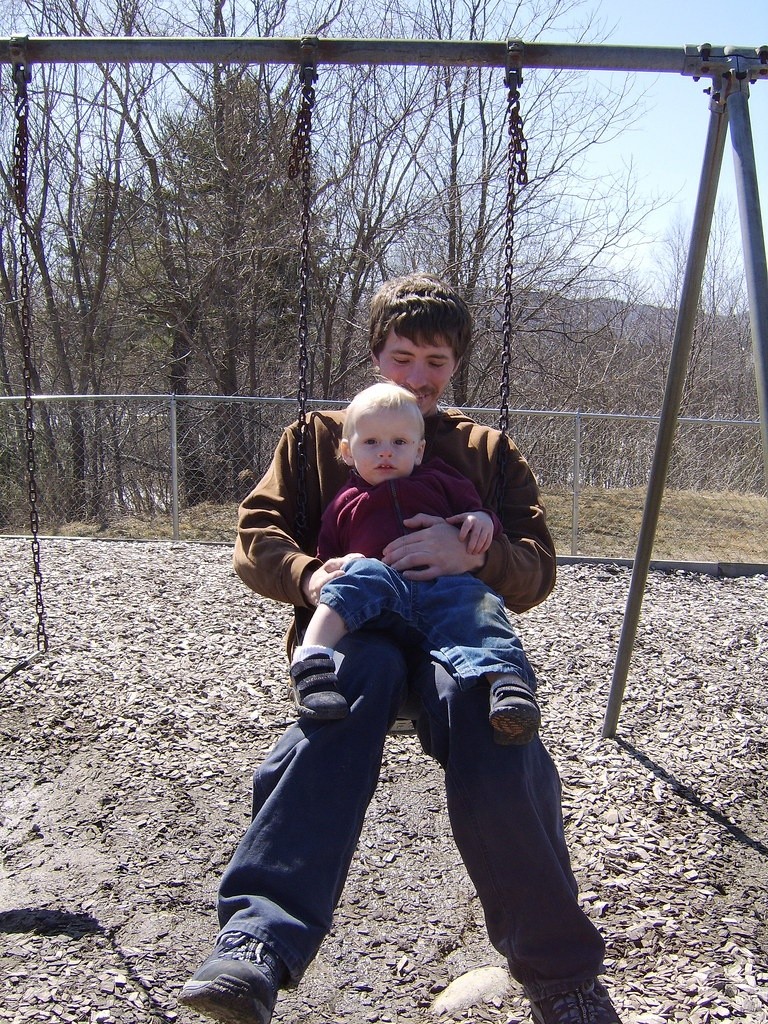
[488, 677, 542, 746]
[178, 930, 285, 1024]
[286, 653, 348, 721]
[524, 977, 623, 1024]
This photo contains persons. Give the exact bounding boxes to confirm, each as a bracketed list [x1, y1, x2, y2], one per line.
[179, 272, 624, 1024]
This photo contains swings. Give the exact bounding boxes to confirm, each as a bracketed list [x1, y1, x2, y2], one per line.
[0, 61, 51, 687]
[292, 63, 526, 742]
